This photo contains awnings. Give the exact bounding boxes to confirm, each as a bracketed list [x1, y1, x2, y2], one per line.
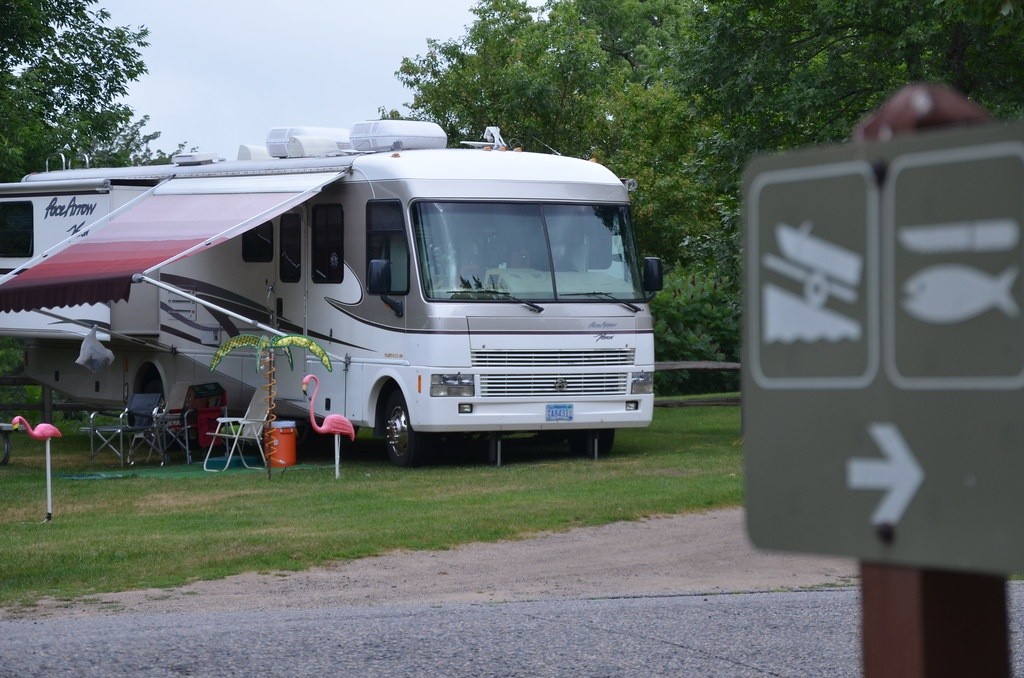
[0, 172, 346, 364]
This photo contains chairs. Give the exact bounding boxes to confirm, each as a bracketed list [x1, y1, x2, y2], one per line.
[203, 382, 278, 472]
[89, 393, 163, 470]
[161, 409, 195, 465]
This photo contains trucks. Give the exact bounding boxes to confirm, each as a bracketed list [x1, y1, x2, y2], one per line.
[1, 121, 666, 464]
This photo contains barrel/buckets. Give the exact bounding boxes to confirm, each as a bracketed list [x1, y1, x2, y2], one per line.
[264, 421, 297, 467]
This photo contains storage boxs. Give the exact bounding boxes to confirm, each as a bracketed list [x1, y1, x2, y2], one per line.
[180, 382, 225, 426]
[263, 420, 297, 467]
[196, 407, 221, 447]
